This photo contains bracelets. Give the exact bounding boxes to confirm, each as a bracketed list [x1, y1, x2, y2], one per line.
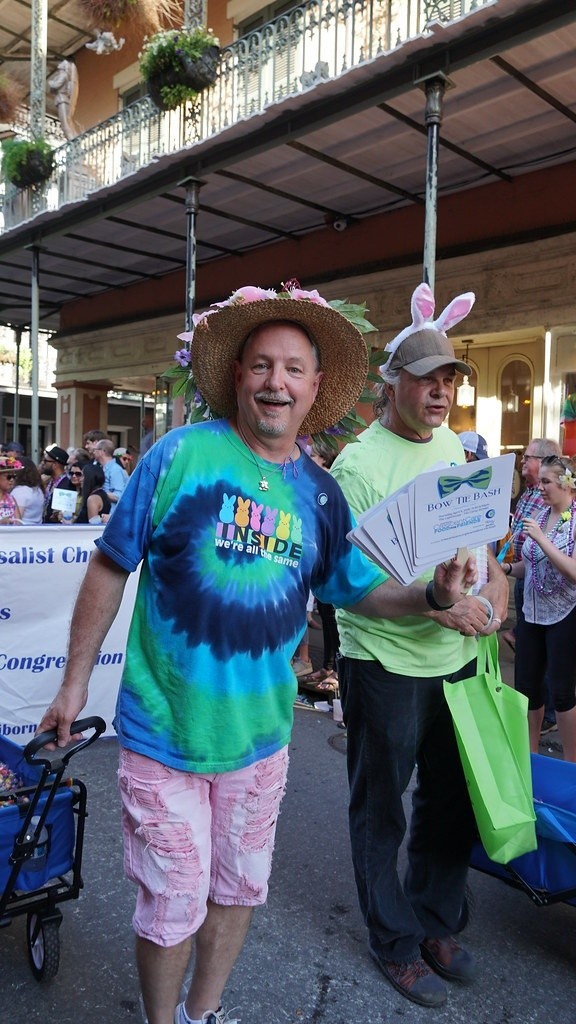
[506, 563, 512, 575]
[425, 579, 456, 611]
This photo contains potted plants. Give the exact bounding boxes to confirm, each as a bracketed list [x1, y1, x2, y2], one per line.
[0, 135, 53, 188]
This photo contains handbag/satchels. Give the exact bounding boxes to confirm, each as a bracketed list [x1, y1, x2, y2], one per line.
[441, 623, 537, 864]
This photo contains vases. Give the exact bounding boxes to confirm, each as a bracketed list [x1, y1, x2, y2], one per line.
[142, 38, 218, 111]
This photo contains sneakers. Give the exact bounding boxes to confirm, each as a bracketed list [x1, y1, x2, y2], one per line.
[420, 934, 479, 985]
[366, 938, 448, 1005]
[540, 720, 558, 734]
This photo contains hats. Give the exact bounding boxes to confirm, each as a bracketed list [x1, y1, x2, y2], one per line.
[45, 446, 70, 466]
[0, 456, 25, 472]
[113, 448, 130, 458]
[388, 329, 472, 377]
[458, 431, 489, 460]
[191, 298, 371, 438]
[4, 442, 22, 451]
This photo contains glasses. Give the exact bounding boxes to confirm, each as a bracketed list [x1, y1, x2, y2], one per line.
[542, 455, 566, 470]
[69, 471, 82, 477]
[93, 448, 105, 452]
[44, 458, 55, 464]
[120, 456, 130, 461]
[1, 474, 17, 480]
[522, 454, 544, 461]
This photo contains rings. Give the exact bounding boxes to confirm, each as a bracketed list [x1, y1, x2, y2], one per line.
[493, 618, 503, 624]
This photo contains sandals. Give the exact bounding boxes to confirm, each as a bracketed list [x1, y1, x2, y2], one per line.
[315, 674, 339, 691]
[298, 670, 328, 683]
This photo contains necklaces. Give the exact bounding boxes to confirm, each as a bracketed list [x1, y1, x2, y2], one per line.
[237, 429, 296, 492]
[531, 501, 575, 596]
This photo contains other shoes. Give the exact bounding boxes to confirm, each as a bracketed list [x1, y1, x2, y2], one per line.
[292, 657, 314, 676]
[175, 999, 241, 1024]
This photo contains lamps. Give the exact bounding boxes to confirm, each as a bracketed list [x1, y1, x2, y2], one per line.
[456, 340, 475, 407]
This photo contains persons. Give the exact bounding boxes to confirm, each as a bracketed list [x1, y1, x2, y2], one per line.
[37, 284, 479, 1023]
[326, 282, 507, 1005]
[0, 430, 136, 524]
[137, 415, 154, 459]
[291, 590, 339, 711]
[497, 437, 576, 764]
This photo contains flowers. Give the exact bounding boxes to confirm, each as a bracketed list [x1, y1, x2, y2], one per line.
[0, 456, 22, 470]
[561, 511, 571, 521]
[135, 23, 221, 77]
[556, 467, 576, 489]
[158, 284, 349, 442]
